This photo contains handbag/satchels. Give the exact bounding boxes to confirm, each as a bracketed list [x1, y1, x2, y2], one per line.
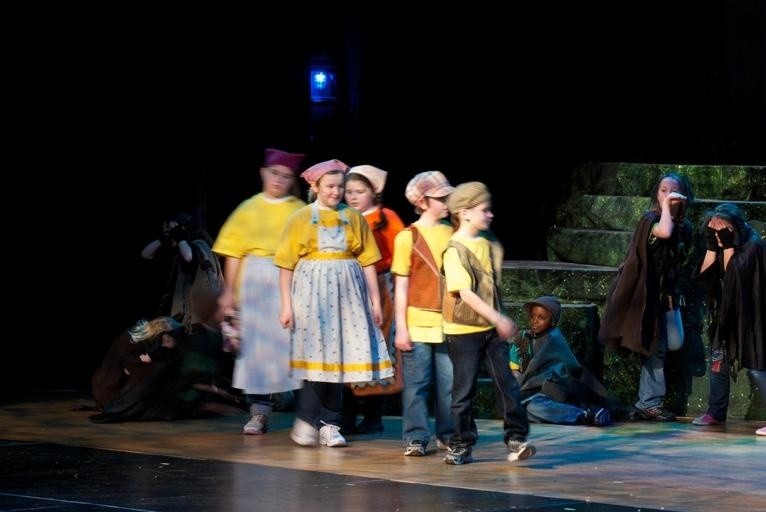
[667, 295, 706, 377]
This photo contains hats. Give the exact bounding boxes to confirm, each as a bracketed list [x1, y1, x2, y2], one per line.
[446, 181, 492, 215]
[523, 296, 561, 322]
[405, 171, 455, 206]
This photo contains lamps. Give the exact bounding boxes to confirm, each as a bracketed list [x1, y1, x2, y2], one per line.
[309, 66, 337, 103]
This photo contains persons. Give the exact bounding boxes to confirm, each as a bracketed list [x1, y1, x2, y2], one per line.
[211, 150, 308, 435]
[273, 160, 394, 446]
[599, 173, 707, 421]
[391, 171, 454, 457]
[442, 182, 538, 465]
[345, 163, 405, 432]
[141, 214, 224, 330]
[89, 315, 180, 417]
[518, 295, 635, 426]
[692, 205, 766, 433]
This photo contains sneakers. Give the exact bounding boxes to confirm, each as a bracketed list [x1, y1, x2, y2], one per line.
[507, 440, 536, 462]
[436, 438, 473, 465]
[290, 417, 384, 447]
[584, 408, 610, 426]
[755, 427, 766, 435]
[243, 414, 269, 435]
[631, 406, 676, 422]
[404, 440, 425, 456]
[692, 413, 719, 425]
